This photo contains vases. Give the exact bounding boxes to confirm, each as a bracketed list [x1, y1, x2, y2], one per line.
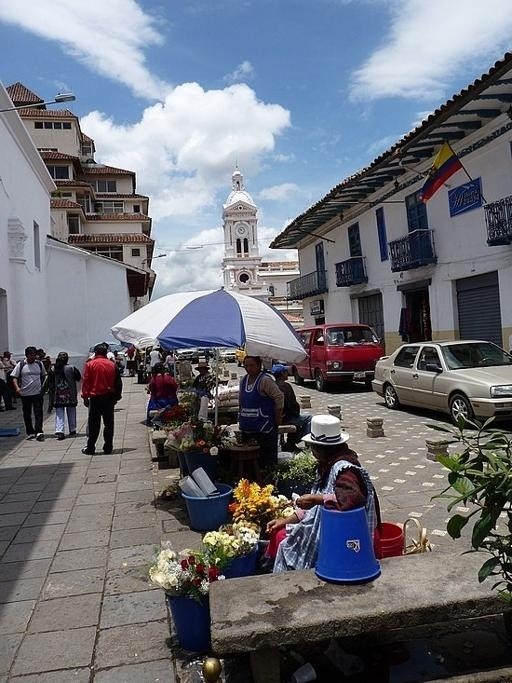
[158, 404, 188, 429]
[163, 417, 236, 456]
[228, 476, 298, 526]
[201, 520, 260, 573]
[147, 547, 227, 602]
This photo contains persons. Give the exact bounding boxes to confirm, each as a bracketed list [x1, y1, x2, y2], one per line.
[10, 347, 48, 440]
[163, 351, 178, 376]
[36, 349, 51, 362]
[238, 356, 284, 473]
[144, 363, 179, 425]
[3, 351, 14, 373]
[86, 341, 116, 364]
[44, 359, 56, 414]
[271, 364, 313, 451]
[125, 344, 137, 377]
[0, 356, 17, 412]
[265, 415, 381, 573]
[49, 352, 82, 438]
[149, 347, 163, 367]
[346, 330, 366, 342]
[114, 350, 125, 373]
[192, 363, 220, 417]
[9, 351, 18, 404]
[81, 344, 122, 456]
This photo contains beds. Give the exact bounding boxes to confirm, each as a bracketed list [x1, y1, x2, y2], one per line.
[110, 285, 309, 428]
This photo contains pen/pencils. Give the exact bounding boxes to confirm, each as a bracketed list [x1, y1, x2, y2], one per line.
[292, 322, 380, 392]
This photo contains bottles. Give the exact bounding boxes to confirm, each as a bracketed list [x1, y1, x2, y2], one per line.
[3, 351, 12, 357]
[195, 361, 210, 370]
[153, 345, 160, 350]
[301, 414, 350, 446]
[270, 364, 287, 373]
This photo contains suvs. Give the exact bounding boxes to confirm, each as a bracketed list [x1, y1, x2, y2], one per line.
[164, 593, 211, 651]
[182, 483, 233, 529]
[212, 544, 259, 580]
[315, 504, 382, 583]
[374, 522, 405, 558]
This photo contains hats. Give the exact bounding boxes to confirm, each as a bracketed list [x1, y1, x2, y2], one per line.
[370, 339, 511, 426]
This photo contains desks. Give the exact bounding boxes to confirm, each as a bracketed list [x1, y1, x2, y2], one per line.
[142, 253, 167, 267]
[0, 91, 78, 114]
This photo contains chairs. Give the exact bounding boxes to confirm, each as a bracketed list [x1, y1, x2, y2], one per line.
[68, 432, 76, 438]
[104, 448, 112, 455]
[81, 446, 95, 455]
[36, 433, 45, 441]
[57, 434, 65, 440]
[25, 434, 35, 441]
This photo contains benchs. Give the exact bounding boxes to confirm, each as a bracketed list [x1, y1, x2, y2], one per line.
[420, 143, 462, 203]
